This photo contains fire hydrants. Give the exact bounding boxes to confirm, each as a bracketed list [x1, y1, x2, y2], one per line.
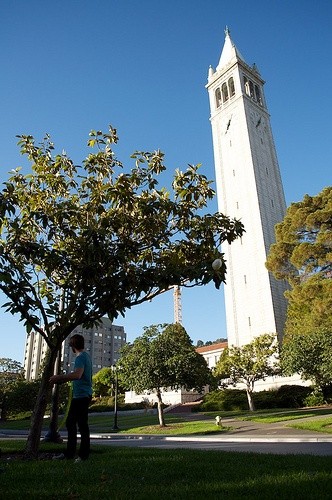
[215, 415, 222, 427]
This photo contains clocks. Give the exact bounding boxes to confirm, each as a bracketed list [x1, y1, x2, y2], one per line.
[249, 109, 268, 137]
[220, 112, 240, 138]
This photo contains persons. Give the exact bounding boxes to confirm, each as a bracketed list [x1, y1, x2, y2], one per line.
[47, 334, 94, 465]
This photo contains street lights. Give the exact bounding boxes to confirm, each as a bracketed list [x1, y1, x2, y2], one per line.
[111, 363, 123, 430]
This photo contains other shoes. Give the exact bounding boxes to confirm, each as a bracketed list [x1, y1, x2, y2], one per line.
[52, 452, 74, 460]
[72, 457, 89, 464]
